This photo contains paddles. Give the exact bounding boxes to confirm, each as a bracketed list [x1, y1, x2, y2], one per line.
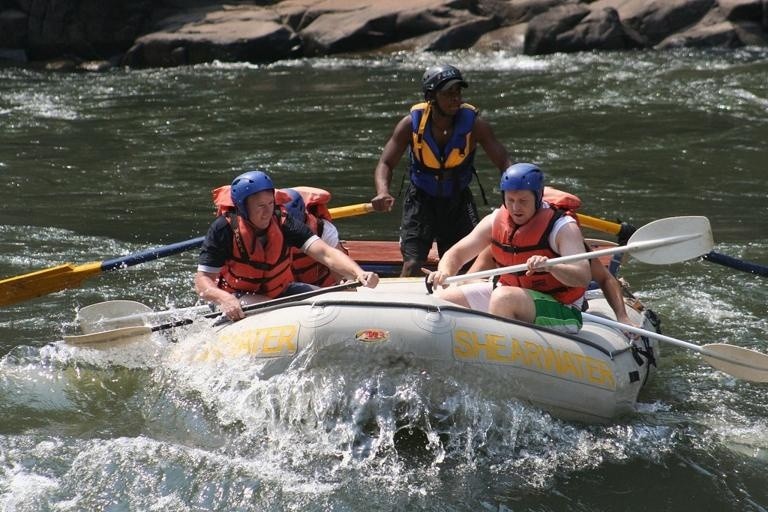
[441, 215, 714, 286]
[0, 203, 375, 309]
[78, 300, 222, 334]
[64, 278, 366, 349]
[582, 312, 768, 384]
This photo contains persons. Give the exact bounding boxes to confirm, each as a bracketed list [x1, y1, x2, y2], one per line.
[196, 170, 380, 322]
[459, 185, 642, 341]
[370, 62, 516, 278]
[276, 189, 351, 288]
[420, 163, 592, 335]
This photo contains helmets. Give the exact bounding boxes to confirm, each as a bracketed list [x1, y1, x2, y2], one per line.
[421, 64, 468, 105]
[280, 188, 305, 223]
[230, 171, 275, 219]
[499, 162, 543, 208]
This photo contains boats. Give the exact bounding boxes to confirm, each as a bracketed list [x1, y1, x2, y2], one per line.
[161, 236, 660, 429]
